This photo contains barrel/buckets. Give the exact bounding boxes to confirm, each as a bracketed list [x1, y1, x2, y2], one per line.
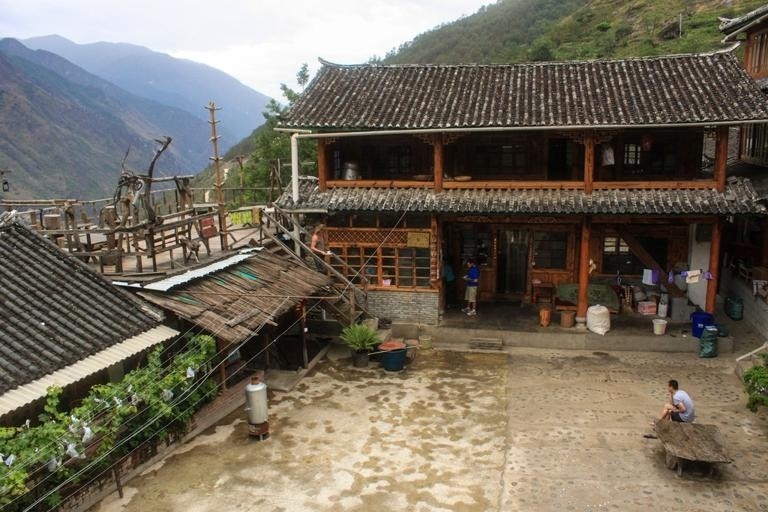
[379, 344, 408, 371]
[652, 319, 667, 334]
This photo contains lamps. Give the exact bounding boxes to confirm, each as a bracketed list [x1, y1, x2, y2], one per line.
[0, 170, 10, 192]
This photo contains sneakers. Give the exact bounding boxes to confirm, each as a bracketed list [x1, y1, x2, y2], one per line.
[466, 309, 477, 316]
[461, 307, 470, 312]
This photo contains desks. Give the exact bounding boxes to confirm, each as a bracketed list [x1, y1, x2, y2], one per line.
[532, 282, 553, 304]
[72, 235, 119, 264]
[654, 420, 731, 476]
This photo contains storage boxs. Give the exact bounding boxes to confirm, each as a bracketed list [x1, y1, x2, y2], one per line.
[639, 301, 656, 315]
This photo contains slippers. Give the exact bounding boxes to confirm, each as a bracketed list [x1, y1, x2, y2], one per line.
[643, 433, 658, 440]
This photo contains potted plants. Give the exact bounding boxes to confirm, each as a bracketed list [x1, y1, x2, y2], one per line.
[339, 324, 380, 368]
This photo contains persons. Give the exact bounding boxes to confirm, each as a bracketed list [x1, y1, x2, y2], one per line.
[310, 224, 333, 294]
[598, 141, 615, 182]
[649, 379, 696, 434]
[498, 240, 509, 293]
[341, 155, 359, 180]
[381, 271, 393, 285]
[441, 257, 460, 311]
[365, 261, 377, 285]
[534, 242, 552, 268]
[461, 258, 480, 315]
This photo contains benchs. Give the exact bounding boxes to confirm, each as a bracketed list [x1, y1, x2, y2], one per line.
[131, 208, 198, 257]
